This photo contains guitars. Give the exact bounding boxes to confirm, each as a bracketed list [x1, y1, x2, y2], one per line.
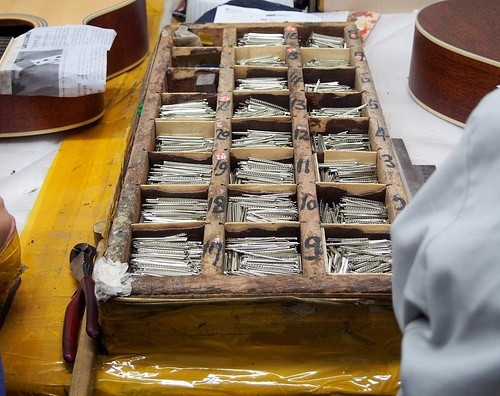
[1, 0, 150, 139]
[407, 0, 500, 129]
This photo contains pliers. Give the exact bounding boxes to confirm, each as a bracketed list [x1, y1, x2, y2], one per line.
[62, 243, 101, 363]
